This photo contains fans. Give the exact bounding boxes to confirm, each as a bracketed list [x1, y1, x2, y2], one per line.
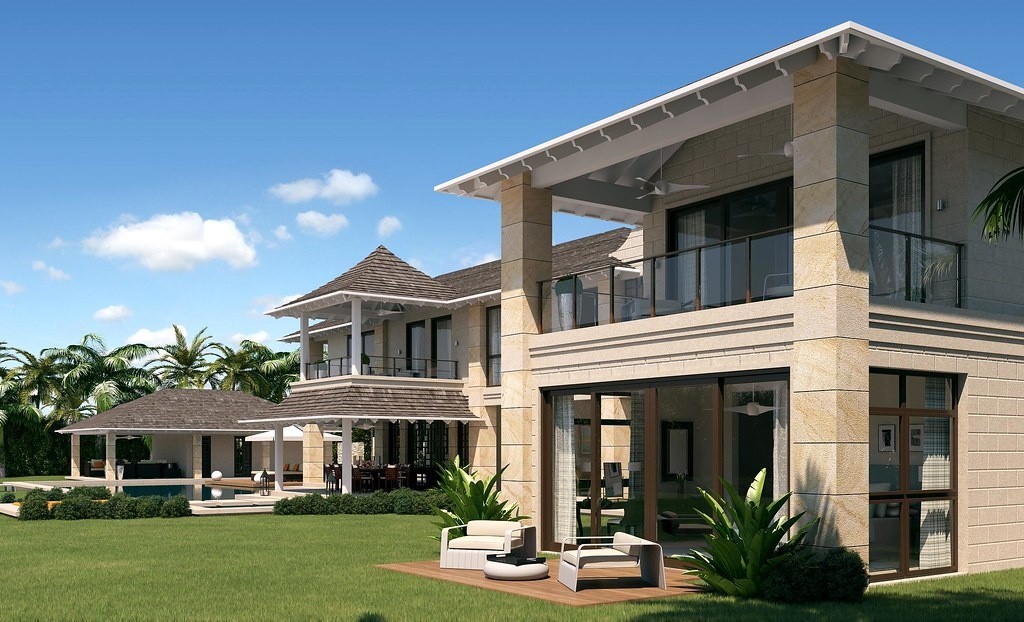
[634, 149, 709, 200]
[737, 104, 794, 158]
[704, 384, 785, 417]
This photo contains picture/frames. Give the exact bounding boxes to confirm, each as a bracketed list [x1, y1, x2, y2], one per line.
[878, 423, 895, 453]
[909, 424, 925, 452]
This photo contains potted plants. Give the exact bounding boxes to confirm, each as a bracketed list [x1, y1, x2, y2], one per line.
[116, 459, 124, 481]
[361, 353, 370, 375]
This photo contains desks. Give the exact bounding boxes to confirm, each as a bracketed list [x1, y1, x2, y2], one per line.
[351, 467, 404, 490]
[577, 497, 626, 534]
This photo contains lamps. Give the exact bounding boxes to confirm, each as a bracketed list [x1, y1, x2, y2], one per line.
[936, 200, 947, 211]
[454, 341, 458, 346]
[655, 261, 660, 269]
[398, 350, 401, 355]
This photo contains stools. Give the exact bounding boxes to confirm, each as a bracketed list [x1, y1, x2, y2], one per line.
[484, 563, 552, 580]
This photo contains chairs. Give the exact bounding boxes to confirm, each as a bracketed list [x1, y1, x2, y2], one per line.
[271, 463, 288, 481]
[630, 299, 681, 319]
[607, 499, 644, 542]
[290, 464, 299, 482]
[439, 521, 536, 568]
[659, 511, 718, 537]
[87, 459, 106, 477]
[557, 532, 668, 593]
[762, 273, 795, 299]
[323, 462, 435, 493]
[395, 366, 410, 377]
